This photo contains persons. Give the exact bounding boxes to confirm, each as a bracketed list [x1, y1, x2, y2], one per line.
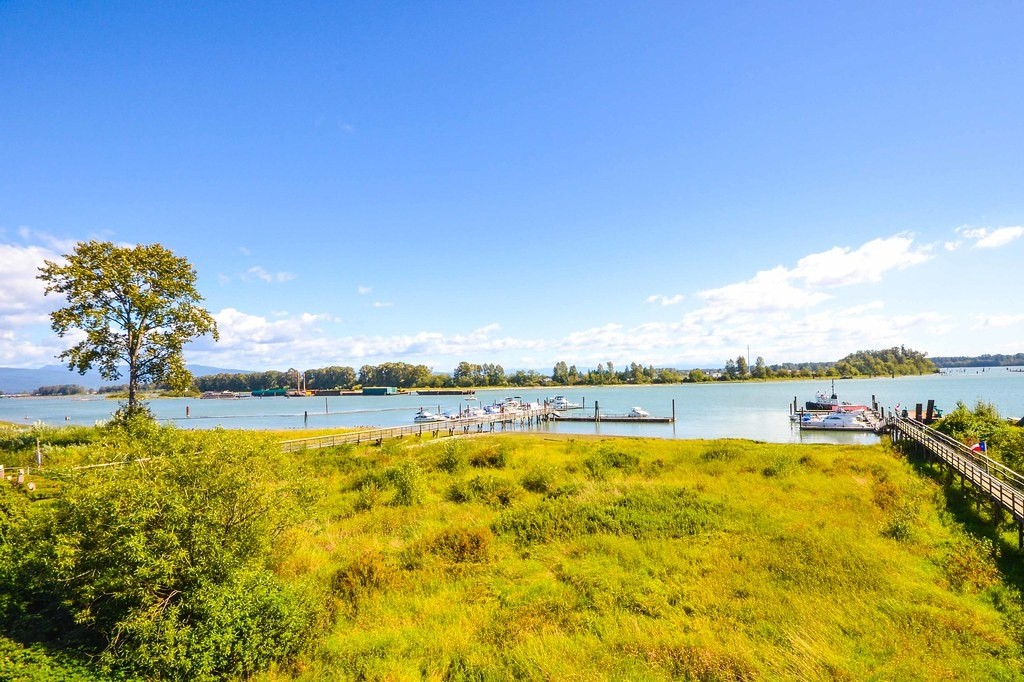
[500, 403, 504, 413]
[895, 404, 900, 417]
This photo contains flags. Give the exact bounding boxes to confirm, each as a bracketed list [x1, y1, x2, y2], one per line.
[970, 442, 986, 454]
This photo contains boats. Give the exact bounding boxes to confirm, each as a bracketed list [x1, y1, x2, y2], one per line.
[465, 395, 477, 401]
[806, 379, 868, 411]
[627, 407, 650, 417]
[473, 407, 486, 415]
[434, 405, 448, 420]
[549, 395, 577, 409]
[801, 415, 864, 427]
[486, 397, 541, 414]
[413, 407, 437, 422]
